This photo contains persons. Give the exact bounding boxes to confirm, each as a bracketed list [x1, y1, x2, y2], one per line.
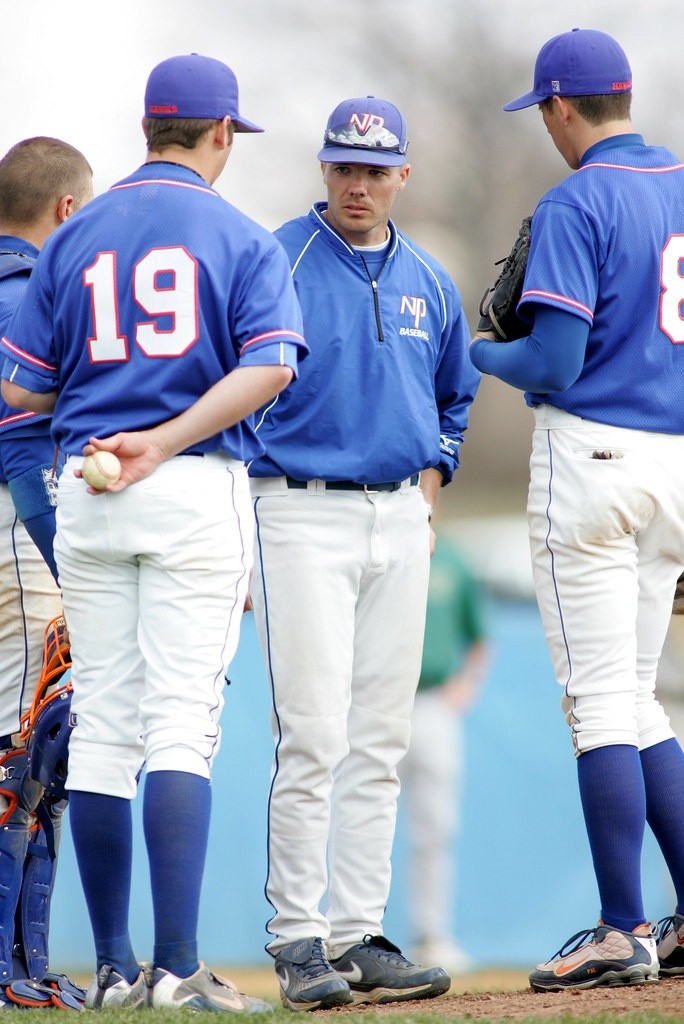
[469, 29, 684, 991]
[0, 51, 310, 1015]
[249, 96, 482, 1009]
[404, 520, 490, 972]
[0, 141, 91, 1011]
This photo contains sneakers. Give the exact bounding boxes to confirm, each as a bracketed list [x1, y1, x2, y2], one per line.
[150, 959, 273, 1016]
[529, 919, 660, 992]
[330, 934, 451, 1006]
[84, 961, 154, 1012]
[652, 906, 684, 977]
[274, 937, 354, 1013]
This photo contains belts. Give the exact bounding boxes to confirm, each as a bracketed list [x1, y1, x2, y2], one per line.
[285, 474, 419, 493]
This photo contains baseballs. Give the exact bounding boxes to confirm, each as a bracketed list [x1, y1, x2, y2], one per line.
[79, 452, 123, 488]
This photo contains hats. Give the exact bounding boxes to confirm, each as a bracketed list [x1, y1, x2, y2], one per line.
[503, 27, 632, 111]
[144, 54, 266, 133]
[317, 96, 409, 167]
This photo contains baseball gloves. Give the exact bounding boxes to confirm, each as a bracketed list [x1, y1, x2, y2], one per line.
[480, 214, 545, 337]
